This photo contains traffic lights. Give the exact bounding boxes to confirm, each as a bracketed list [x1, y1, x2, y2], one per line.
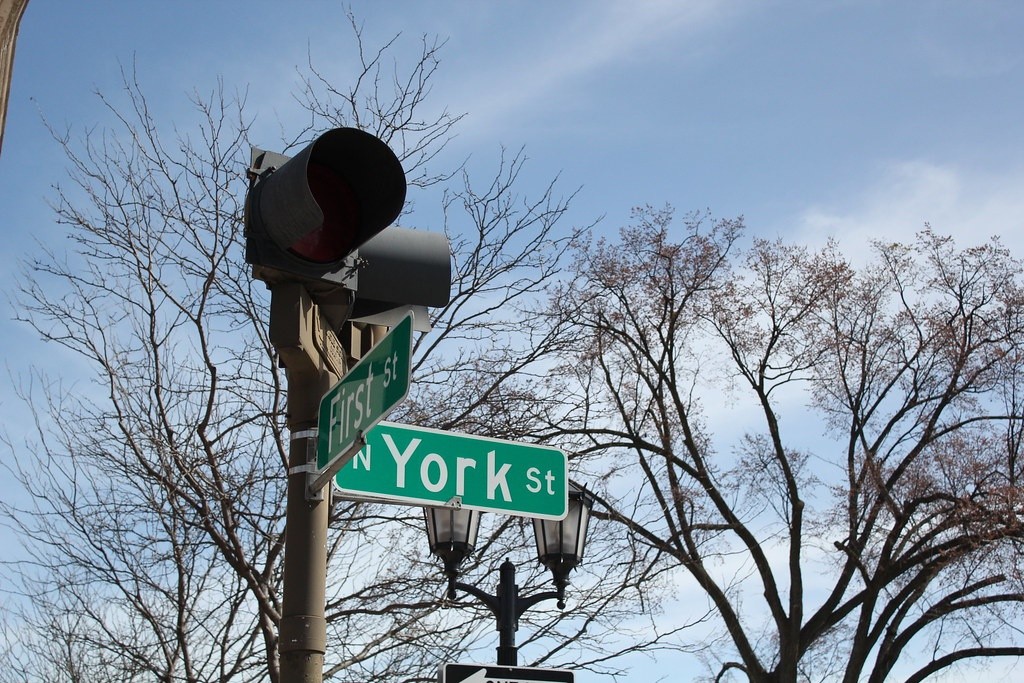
[354, 225, 453, 332]
[247, 126, 408, 293]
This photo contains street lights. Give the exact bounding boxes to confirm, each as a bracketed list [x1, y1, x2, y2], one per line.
[423, 477, 598, 666]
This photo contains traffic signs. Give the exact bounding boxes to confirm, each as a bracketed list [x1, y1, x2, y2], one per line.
[443, 663, 575, 683]
